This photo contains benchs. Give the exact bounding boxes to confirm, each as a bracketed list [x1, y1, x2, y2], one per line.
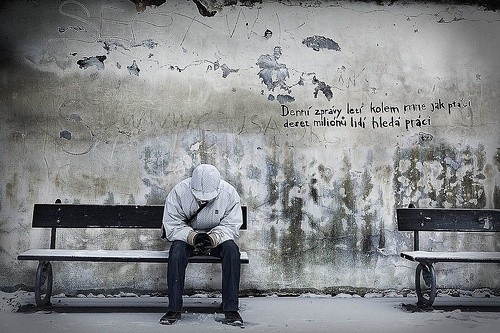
[396, 208, 500, 312]
[17, 198, 249, 314]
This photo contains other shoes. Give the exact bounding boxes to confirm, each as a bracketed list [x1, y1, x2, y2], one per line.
[225, 309, 244, 326]
[159, 308, 182, 325]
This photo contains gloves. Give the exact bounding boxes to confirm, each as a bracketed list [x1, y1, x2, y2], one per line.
[193, 232, 213, 246]
[192, 244, 212, 255]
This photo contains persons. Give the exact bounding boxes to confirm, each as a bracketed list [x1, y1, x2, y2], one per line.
[160, 163, 244, 327]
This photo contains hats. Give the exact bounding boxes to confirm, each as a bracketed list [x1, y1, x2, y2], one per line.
[191, 164, 221, 201]
